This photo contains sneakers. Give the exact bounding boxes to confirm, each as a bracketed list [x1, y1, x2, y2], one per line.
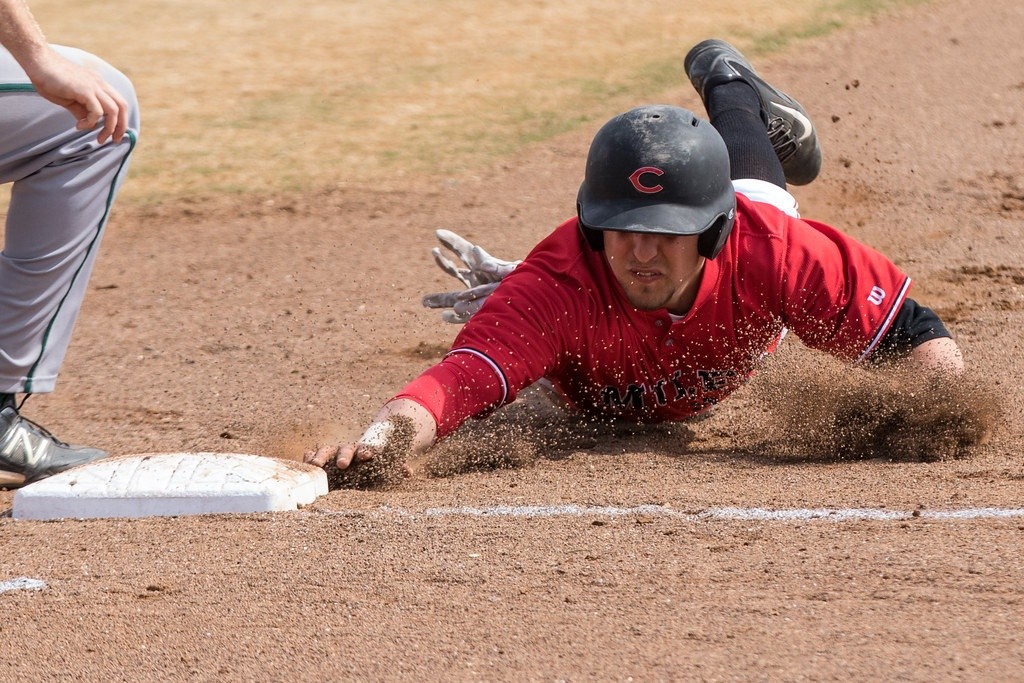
[684, 38, 822, 187]
[0, 393, 107, 487]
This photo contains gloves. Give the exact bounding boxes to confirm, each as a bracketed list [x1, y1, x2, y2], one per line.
[420, 228, 523, 324]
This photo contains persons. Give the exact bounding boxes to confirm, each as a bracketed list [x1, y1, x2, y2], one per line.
[301, 37, 963, 476]
[0, 1, 140, 489]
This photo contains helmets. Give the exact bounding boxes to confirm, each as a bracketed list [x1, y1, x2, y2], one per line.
[577, 104, 737, 260]
